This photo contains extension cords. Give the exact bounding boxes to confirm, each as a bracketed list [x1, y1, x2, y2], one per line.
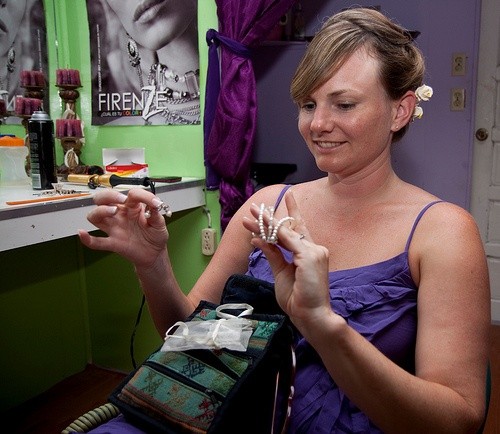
[202, 228, 217, 256]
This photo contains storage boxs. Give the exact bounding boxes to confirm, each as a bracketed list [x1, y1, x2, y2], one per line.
[102, 148, 148, 179]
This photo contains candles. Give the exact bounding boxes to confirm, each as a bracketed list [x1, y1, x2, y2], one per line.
[15, 69, 83, 137]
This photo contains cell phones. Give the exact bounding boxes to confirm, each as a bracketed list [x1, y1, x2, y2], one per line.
[148, 174, 182, 182]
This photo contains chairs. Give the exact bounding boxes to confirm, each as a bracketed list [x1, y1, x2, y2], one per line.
[61, 350, 492, 434]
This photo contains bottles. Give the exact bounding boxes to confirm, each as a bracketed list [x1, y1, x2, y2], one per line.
[28, 107, 57, 190]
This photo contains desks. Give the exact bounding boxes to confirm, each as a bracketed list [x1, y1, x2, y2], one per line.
[0, 176, 206, 263]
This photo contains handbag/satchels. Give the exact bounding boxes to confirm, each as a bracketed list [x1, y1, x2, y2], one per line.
[107, 274, 304, 434]
